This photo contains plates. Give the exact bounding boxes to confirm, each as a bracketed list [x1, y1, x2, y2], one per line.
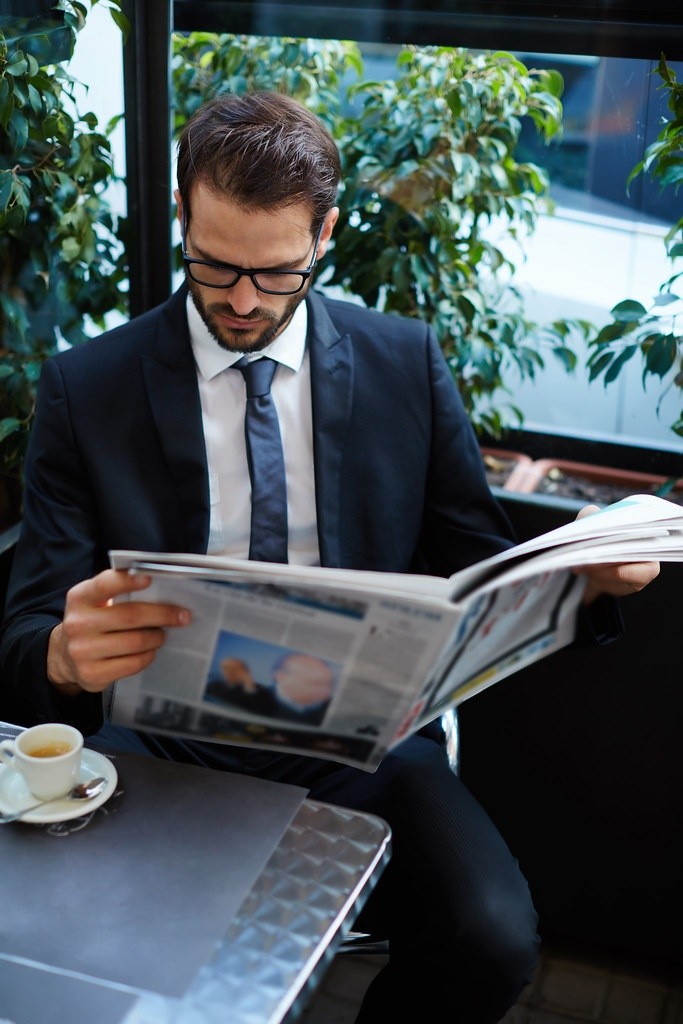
[0, 748, 118, 822]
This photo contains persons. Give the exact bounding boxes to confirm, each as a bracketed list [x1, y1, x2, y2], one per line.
[202, 653, 334, 728]
[0, 92, 661, 1024]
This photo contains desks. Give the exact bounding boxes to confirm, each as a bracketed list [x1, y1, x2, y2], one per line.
[0, 720, 392, 1024]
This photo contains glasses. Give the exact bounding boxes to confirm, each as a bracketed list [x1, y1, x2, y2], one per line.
[181, 200, 325, 296]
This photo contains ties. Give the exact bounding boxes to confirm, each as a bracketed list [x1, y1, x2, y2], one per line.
[228, 356, 288, 563]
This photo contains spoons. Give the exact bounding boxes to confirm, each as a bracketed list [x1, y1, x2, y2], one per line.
[0, 778, 107, 823]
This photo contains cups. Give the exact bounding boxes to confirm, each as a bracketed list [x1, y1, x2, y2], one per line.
[0, 723, 83, 801]
